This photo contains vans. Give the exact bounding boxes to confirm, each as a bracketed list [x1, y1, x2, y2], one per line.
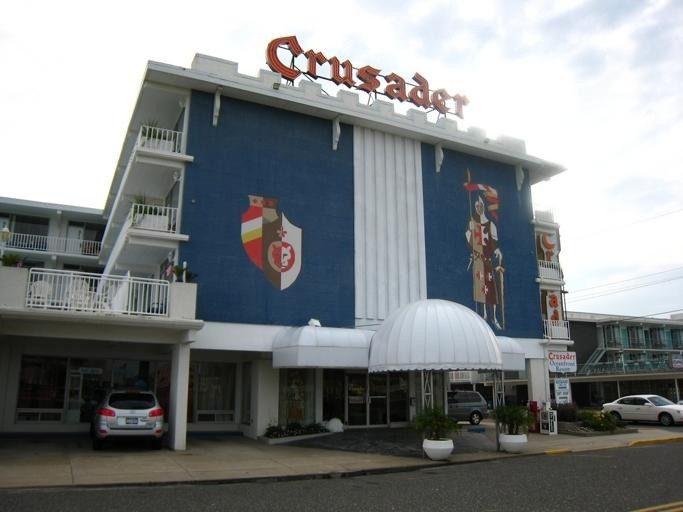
[447, 391, 488, 425]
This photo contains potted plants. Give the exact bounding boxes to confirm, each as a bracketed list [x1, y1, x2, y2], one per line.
[411, 405, 461, 460]
[492, 401, 534, 454]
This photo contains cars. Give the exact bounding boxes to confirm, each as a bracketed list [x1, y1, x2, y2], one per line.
[90, 388, 166, 451]
[600, 394, 683, 426]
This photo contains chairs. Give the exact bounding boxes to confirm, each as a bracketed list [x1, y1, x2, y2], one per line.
[29, 279, 91, 311]
